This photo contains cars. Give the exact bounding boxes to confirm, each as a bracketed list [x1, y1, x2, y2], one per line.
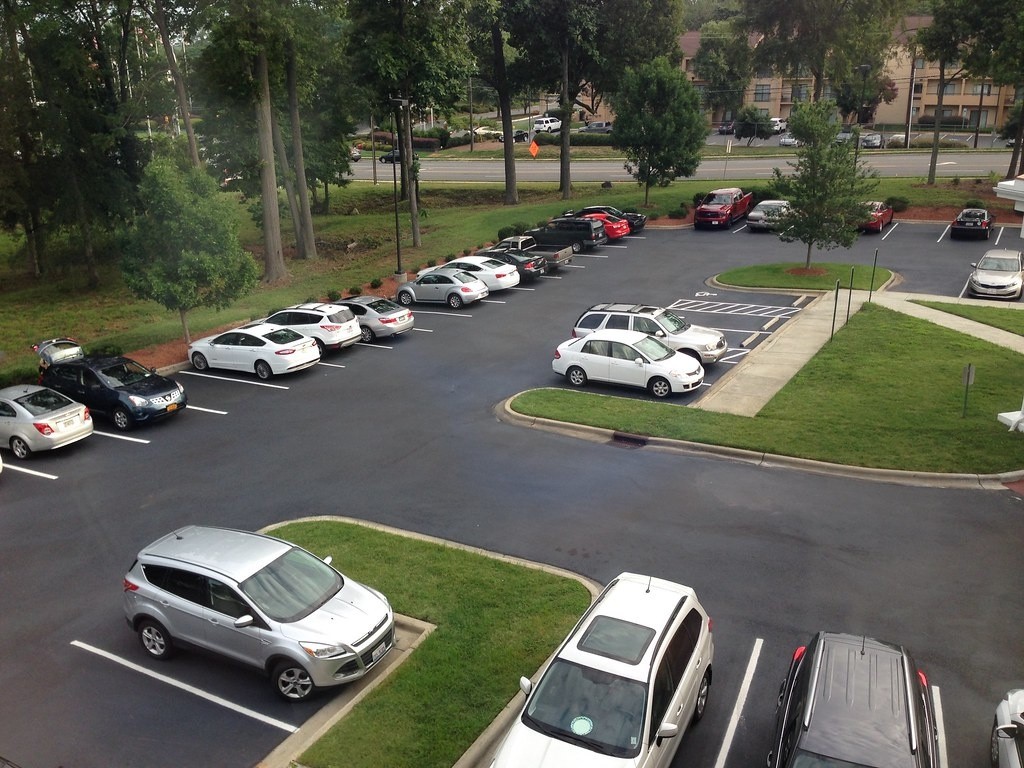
[856, 201, 893, 234]
[473, 125, 504, 138]
[349, 148, 362, 162]
[989, 688, 1024, 768]
[718, 123, 734, 135]
[950, 209, 997, 240]
[583, 213, 631, 245]
[862, 134, 881, 149]
[475, 249, 549, 286]
[835, 133, 853, 145]
[777, 133, 805, 147]
[552, 328, 705, 399]
[888, 134, 906, 146]
[396, 267, 490, 310]
[334, 296, 415, 344]
[414, 256, 521, 294]
[747, 200, 790, 233]
[967, 248, 1024, 301]
[0, 384, 95, 463]
[498, 129, 528, 143]
[1006, 138, 1024, 148]
[378, 149, 419, 164]
[187, 323, 321, 381]
[573, 205, 647, 236]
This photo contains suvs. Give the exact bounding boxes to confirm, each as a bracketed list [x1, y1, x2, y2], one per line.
[34, 335, 188, 432]
[123, 524, 398, 702]
[533, 116, 562, 134]
[489, 571, 715, 768]
[767, 628, 938, 768]
[521, 217, 608, 255]
[570, 302, 728, 368]
[769, 117, 787, 135]
[253, 303, 362, 360]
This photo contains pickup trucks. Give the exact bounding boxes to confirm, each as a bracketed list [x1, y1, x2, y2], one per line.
[490, 236, 573, 272]
[578, 121, 613, 134]
[694, 188, 752, 231]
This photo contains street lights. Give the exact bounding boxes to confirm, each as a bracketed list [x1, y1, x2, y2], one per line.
[387, 93, 409, 275]
[851, 63, 871, 191]
